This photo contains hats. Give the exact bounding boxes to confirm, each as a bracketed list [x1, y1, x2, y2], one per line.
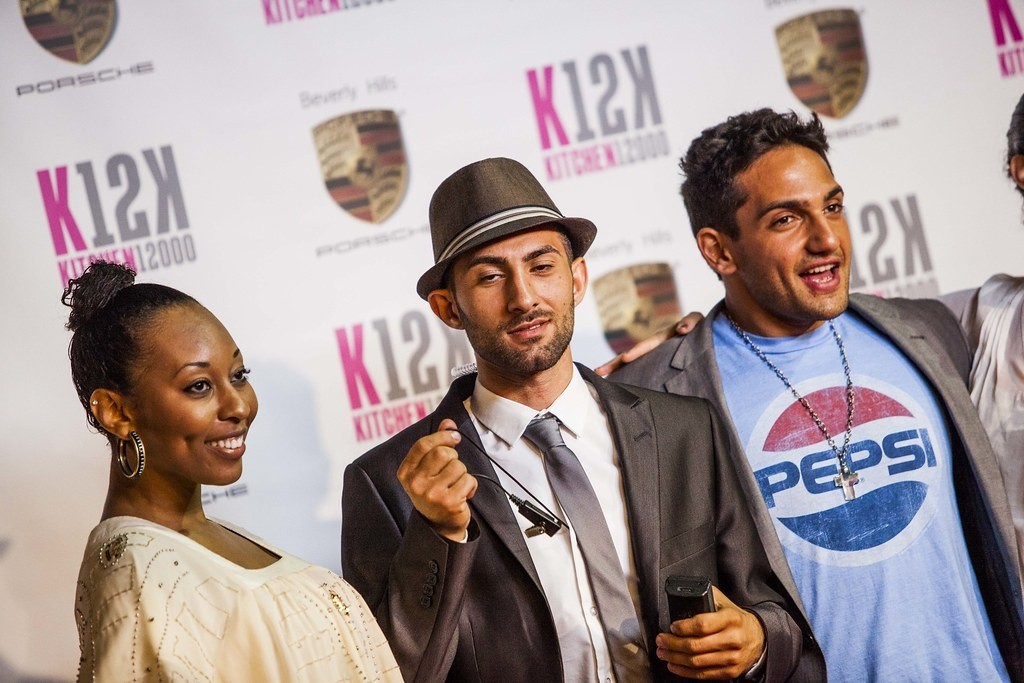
[417, 155, 596, 301]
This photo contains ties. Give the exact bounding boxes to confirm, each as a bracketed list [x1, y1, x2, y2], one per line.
[525, 413, 655, 683]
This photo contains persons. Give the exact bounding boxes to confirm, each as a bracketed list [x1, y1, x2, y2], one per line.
[593, 93, 1024, 564]
[342, 158, 804, 683]
[606, 109, 1024, 683]
[63, 261, 404, 683]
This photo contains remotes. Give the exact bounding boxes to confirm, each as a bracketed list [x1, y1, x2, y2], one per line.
[665, 575, 717, 633]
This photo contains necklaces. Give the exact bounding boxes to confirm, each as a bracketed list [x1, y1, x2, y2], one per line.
[730, 313, 859, 501]
